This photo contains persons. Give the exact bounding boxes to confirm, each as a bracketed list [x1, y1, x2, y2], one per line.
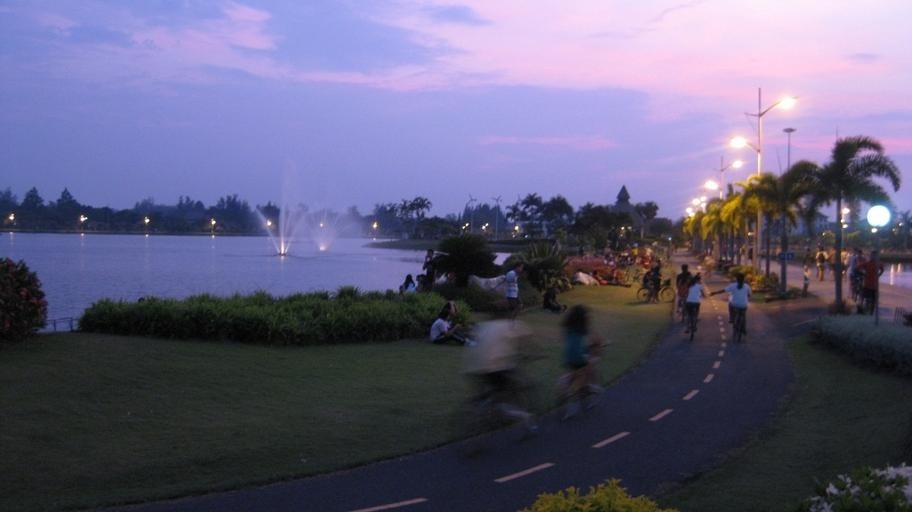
[800, 265, 812, 298]
[453, 299, 546, 460]
[801, 245, 850, 282]
[584, 268, 617, 286]
[846, 247, 885, 316]
[671, 260, 710, 334]
[557, 303, 607, 425]
[398, 249, 435, 295]
[545, 285, 567, 312]
[504, 262, 524, 321]
[431, 300, 477, 348]
[710, 269, 755, 335]
[644, 258, 666, 308]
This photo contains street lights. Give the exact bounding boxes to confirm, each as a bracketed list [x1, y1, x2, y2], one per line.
[866, 205, 890, 325]
[841, 208, 850, 247]
[685, 86, 796, 291]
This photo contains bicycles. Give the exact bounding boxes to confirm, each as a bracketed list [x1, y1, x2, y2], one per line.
[442, 339, 615, 463]
[733, 309, 744, 341]
[689, 315, 700, 341]
[637, 279, 676, 303]
